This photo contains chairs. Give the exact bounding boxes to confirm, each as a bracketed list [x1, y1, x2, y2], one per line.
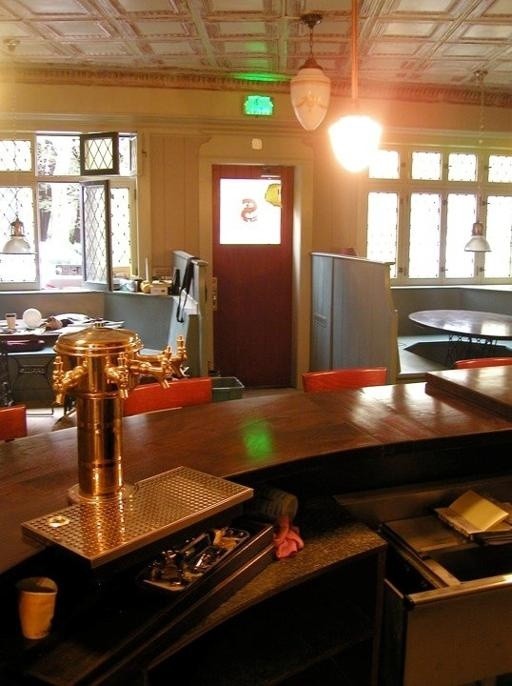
[122, 378, 212, 417]
[390, 284, 512, 383]
[302, 367, 388, 392]
[454, 357, 512, 369]
[0, 406, 27, 440]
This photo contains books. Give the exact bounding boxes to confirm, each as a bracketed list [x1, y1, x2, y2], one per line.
[383, 488, 512, 561]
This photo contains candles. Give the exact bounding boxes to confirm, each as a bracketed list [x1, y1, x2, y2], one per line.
[145, 257, 149, 281]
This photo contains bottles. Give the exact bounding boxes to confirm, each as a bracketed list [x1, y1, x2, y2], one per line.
[257, 484, 299, 521]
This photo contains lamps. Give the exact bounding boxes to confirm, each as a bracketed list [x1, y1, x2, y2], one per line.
[328, 0, 383, 174]
[464, 70, 492, 252]
[0, 39, 35, 255]
[290, 13, 331, 131]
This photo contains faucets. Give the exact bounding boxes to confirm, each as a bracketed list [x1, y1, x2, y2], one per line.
[51, 337, 186, 406]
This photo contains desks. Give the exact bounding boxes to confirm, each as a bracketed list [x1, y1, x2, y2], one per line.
[408, 309, 512, 367]
[0, 319, 77, 415]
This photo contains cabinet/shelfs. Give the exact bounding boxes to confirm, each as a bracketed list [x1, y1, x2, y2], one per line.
[148, 507, 389, 686]
[333, 476, 511, 686]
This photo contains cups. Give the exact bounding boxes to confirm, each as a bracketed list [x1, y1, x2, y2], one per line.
[14, 575, 60, 642]
[6, 312, 18, 329]
